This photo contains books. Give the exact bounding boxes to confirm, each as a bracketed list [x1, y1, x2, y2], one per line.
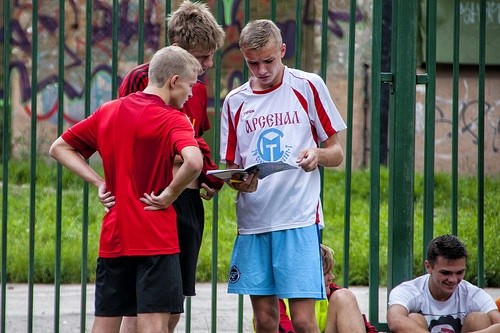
[205, 160, 298, 185]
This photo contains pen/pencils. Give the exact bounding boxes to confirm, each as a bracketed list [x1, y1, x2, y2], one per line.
[226, 179, 244, 183]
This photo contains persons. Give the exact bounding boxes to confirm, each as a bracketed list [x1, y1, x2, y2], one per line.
[220, 20, 381, 333]
[117, 0, 225, 333]
[387, 234, 500, 333]
[48, 45, 204, 333]
